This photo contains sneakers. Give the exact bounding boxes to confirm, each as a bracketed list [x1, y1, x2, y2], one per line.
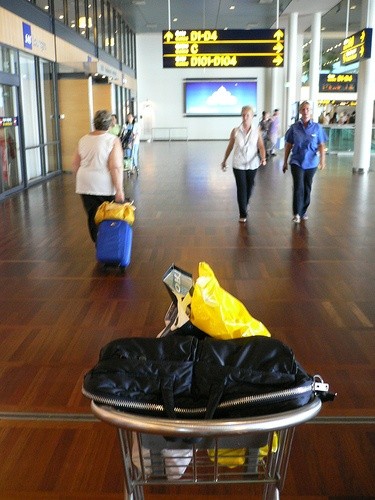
[292, 214, 300, 223]
[239, 218, 247, 223]
[302, 211, 309, 221]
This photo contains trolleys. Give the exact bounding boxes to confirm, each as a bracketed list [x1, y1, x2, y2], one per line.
[119, 129, 139, 177]
[87, 394, 323, 500]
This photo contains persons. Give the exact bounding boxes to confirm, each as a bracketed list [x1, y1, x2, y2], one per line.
[221, 105, 267, 224]
[257, 111, 278, 162]
[318, 110, 356, 124]
[271, 109, 280, 156]
[72, 110, 125, 248]
[283, 100, 328, 223]
[107, 114, 121, 136]
[119, 112, 141, 177]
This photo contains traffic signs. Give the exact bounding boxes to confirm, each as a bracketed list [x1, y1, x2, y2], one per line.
[340, 42, 366, 65]
[162, 30, 284, 42]
[341, 27, 366, 53]
[163, 43, 284, 54]
[164, 54, 284, 68]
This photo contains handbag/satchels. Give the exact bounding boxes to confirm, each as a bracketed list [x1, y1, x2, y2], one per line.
[132, 262, 199, 481]
[191, 260, 279, 469]
[82, 336, 337, 444]
[95, 200, 136, 225]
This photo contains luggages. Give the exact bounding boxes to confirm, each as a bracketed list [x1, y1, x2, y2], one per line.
[96, 198, 132, 272]
